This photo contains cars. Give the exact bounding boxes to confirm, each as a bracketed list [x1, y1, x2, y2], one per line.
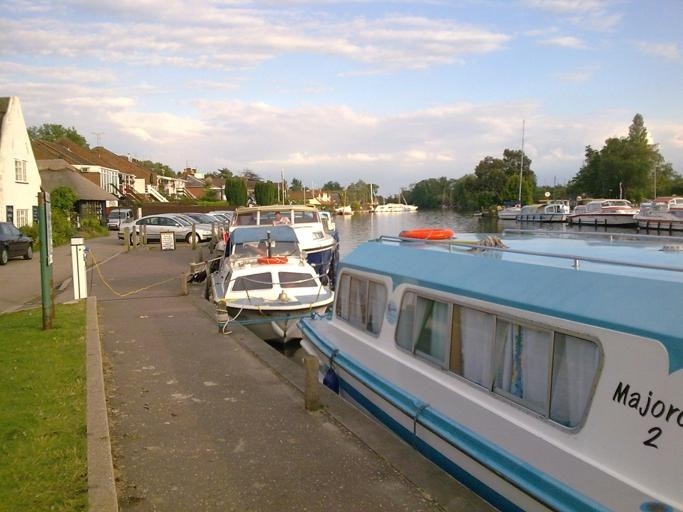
[107, 208, 236, 244]
[0, 222, 33, 265]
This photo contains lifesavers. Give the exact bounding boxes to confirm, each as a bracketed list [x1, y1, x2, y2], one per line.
[257, 256, 288, 265]
[398, 227, 454, 240]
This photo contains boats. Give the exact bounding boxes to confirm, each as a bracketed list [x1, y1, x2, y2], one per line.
[498, 196, 683, 229]
[375, 203, 418, 212]
[208, 203, 339, 343]
[297, 228, 683, 512]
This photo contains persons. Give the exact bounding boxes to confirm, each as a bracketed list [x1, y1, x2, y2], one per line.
[272, 211, 289, 224]
